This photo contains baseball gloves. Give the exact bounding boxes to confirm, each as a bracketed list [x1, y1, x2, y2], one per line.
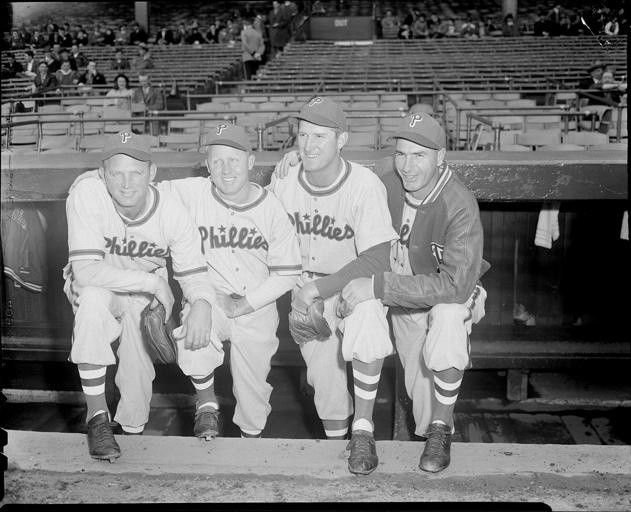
[141, 281, 184, 366]
[289, 297, 328, 345]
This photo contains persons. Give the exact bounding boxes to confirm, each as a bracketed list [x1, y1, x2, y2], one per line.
[62, 131, 216, 462]
[1, 18, 240, 50]
[68, 123, 304, 439]
[261, 95, 400, 476]
[273, 112, 491, 474]
[1, 42, 164, 116]
[239, 1, 304, 76]
[577, 64, 629, 121]
[381, 1, 628, 40]
[311, 1, 352, 16]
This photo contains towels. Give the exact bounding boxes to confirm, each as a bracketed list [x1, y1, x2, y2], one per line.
[620, 211, 629, 240]
[534, 201, 560, 249]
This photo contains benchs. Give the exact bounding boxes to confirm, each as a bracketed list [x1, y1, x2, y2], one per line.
[1, 326, 631, 413]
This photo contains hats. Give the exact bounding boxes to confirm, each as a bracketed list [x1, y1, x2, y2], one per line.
[203, 124, 253, 150]
[295, 99, 347, 128]
[387, 113, 445, 151]
[102, 131, 150, 163]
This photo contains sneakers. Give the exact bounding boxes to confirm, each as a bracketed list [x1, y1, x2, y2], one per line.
[419, 424, 451, 473]
[345, 431, 377, 474]
[87, 412, 120, 460]
[193, 406, 219, 437]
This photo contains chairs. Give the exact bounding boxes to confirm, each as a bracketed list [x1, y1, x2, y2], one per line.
[0, 33, 627, 153]
